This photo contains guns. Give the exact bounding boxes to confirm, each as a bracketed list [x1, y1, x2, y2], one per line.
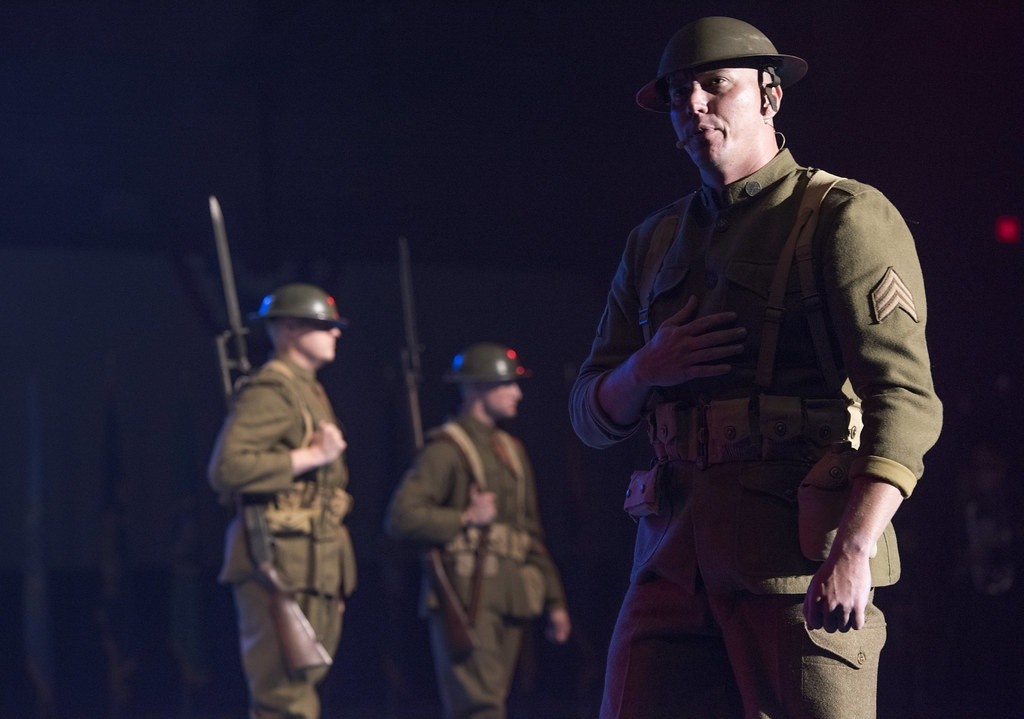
[207, 191, 331, 668]
[393, 231, 481, 658]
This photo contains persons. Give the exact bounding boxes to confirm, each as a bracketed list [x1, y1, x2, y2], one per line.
[210, 285, 356, 719]
[385, 346, 568, 719]
[569, 16, 944, 719]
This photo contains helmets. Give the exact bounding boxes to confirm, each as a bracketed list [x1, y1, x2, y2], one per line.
[251, 284, 350, 329]
[443, 341, 532, 384]
[635, 17, 809, 115]
